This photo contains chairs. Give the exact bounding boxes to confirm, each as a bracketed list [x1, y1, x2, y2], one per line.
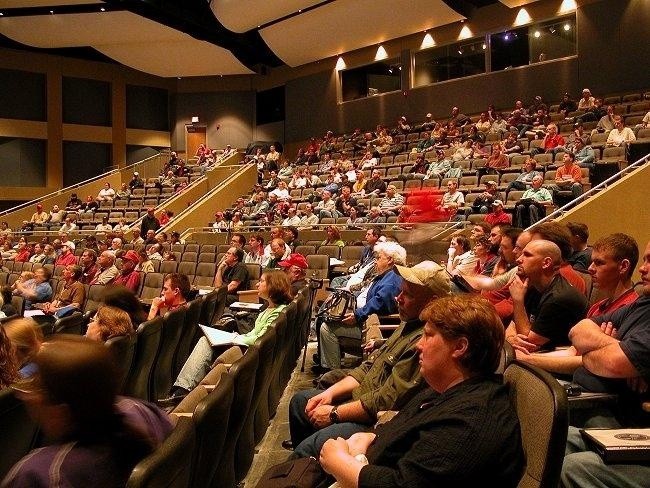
[0, 92, 650, 488]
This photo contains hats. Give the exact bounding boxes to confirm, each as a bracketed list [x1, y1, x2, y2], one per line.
[437, 149, 445, 154]
[103, 216, 109, 220]
[355, 170, 364, 176]
[254, 183, 262, 187]
[216, 212, 223, 217]
[401, 117, 406, 122]
[122, 251, 139, 265]
[426, 113, 432, 118]
[484, 180, 497, 186]
[277, 253, 309, 269]
[392, 261, 452, 298]
[61, 241, 76, 250]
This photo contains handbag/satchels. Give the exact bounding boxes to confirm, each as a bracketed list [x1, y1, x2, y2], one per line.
[254, 456, 328, 488]
[315, 290, 356, 322]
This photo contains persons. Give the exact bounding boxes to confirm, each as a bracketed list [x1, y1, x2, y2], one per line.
[2, 88, 649, 487]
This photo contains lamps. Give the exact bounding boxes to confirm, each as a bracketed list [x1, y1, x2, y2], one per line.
[531, 20, 575, 38]
[458, 41, 486, 54]
[388, 63, 401, 74]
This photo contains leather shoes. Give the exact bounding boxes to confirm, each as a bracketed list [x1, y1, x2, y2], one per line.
[156, 392, 188, 406]
